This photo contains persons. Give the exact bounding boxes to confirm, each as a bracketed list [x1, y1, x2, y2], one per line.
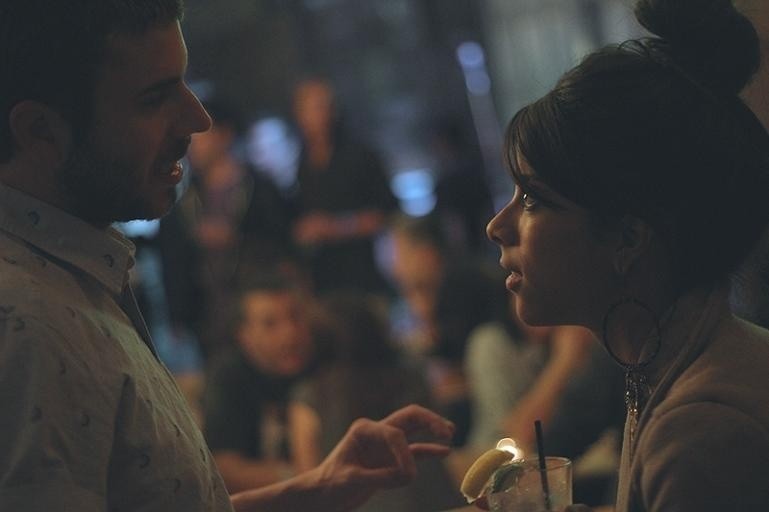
[118, 75, 630, 512]
[2, 0, 460, 512]
[481, 2, 766, 510]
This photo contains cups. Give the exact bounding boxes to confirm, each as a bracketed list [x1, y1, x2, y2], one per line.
[486, 456, 573, 512]
